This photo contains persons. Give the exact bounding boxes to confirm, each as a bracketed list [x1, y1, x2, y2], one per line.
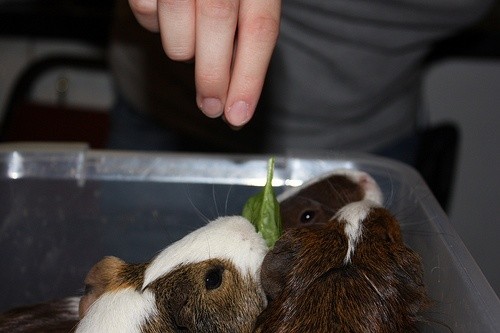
[108, 0, 496, 171]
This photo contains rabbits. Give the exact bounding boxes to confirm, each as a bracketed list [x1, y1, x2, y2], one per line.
[260, 200, 429, 333]
[1, 167, 385, 333]
[72, 215, 266, 333]
